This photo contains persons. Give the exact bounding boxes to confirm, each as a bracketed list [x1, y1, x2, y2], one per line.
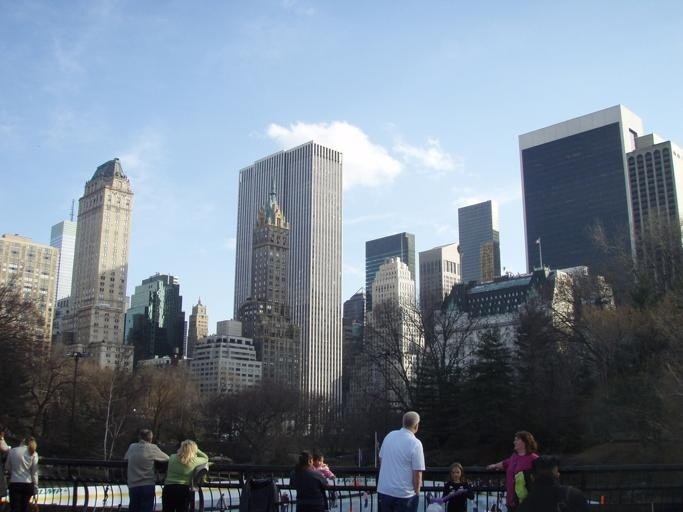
[427, 491, 433, 507]
[486, 431, 540, 512]
[461, 478, 506, 512]
[103, 484, 109, 501]
[514, 455, 590, 512]
[441, 462, 474, 512]
[376, 410, 425, 511]
[290, 450, 329, 512]
[0, 424, 12, 510]
[5, 435, 39, 512]
[280, 492, 289, 512]
[124, 429, 169, 512]
[306, 453, 336, 480]
[216, 493, 228, 512]
[363, 490, 369, 508]
[161, 439, 209, 512]
[330, 491, 338, 509]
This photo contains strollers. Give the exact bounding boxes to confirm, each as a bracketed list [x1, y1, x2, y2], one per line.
[239, 472, 279, 512]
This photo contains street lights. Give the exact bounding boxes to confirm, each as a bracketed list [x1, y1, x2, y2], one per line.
[66, 351, 94, 442]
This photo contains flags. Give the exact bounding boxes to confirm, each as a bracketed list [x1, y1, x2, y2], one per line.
[535, 236, 541, 246]
